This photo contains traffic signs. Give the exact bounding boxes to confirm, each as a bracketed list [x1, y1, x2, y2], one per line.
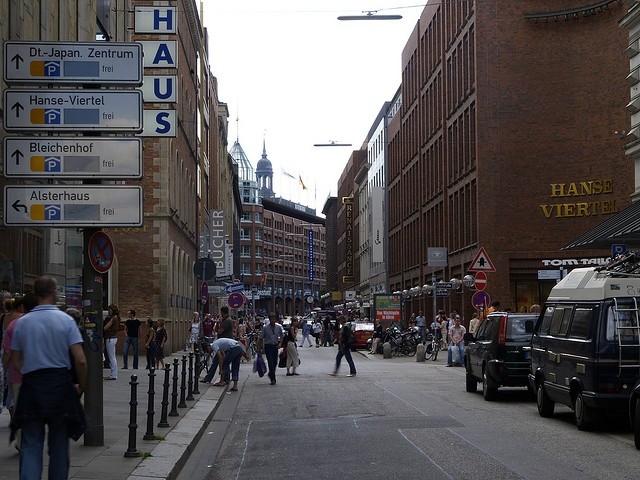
[2, 135, 144, 181]
[3, 185, 144, 227]
[3, 87, 143, 133]
[3, 40, 144, 85]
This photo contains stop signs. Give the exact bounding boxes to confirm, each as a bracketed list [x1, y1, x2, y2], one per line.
[88, 231, 114, 273]
[473, 272, 488, 291]
[228, 293, 243, 309]
[471, 292, 491, 311]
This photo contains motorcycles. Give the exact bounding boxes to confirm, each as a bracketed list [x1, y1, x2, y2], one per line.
[366, 326, 389, 351]
[386, 326, 421, 354]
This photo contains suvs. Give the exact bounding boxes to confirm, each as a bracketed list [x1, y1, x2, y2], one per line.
[464, 311, 541, 401]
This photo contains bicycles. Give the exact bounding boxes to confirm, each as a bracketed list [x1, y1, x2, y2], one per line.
[381, 329, 417, 357]
[199, 332, 217, 377]
[423, 333, 443, 361]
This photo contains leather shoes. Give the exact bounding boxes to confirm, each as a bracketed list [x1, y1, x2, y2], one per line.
[270, 380, 276, 385]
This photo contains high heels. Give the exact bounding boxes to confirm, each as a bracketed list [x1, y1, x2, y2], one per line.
[200, 378, 212, 383]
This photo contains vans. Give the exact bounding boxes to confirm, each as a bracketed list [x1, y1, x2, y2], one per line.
[345, 322, 374, 352]
[525, 267, 640, 431]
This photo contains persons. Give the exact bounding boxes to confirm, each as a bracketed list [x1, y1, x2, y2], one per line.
[11, 276, 90, 476]
[142, 315, 157, 374]
[103, 304, 120, 380]
[158, 320, 168, 367]
[315, 318, 321, 353]
[200, 305, 232, 387]
[448, 314, 464, 367]
[287, 318, 302, 377]
[436, 314, 448, 349]
[369, 318, 380, 356]
[303, 322, 313, 351]
[201, 337, 243, 394]
[413, 308, 430, 344]
[256, 312, 284, 384]
[329, 314, 355, 377]
[122, 309, 139, 372]
[185, 312, 197, 354]
[231, 316, 265, 349]
[323, 317, 333, 351]
[489, 301, 499, 316]
[468, 311, 479, 338]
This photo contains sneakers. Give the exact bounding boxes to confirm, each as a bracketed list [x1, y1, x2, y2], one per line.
[292, 373, 300, 376]
[104, 375, 116, 380]
[309, 345, 313, 347]
[422, 341, 426, 345]
[346, 373, 356, 377]
[230, 387, 238, 391]
[368, 350, 372, 354]
[441, 348, 444, 351]
[461, 364, 465, 367]
[327, 371, 337, 376]
[14, 444, 20, 454]
[324, 344, 328, 347]
[299, 345, 303, 347]
[373, 351, 375, 354]
[286, 373, 294, 376]
[445, 364, 453, 367]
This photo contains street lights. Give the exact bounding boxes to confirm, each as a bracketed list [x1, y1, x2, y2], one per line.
[251, 259, 282, 325]
